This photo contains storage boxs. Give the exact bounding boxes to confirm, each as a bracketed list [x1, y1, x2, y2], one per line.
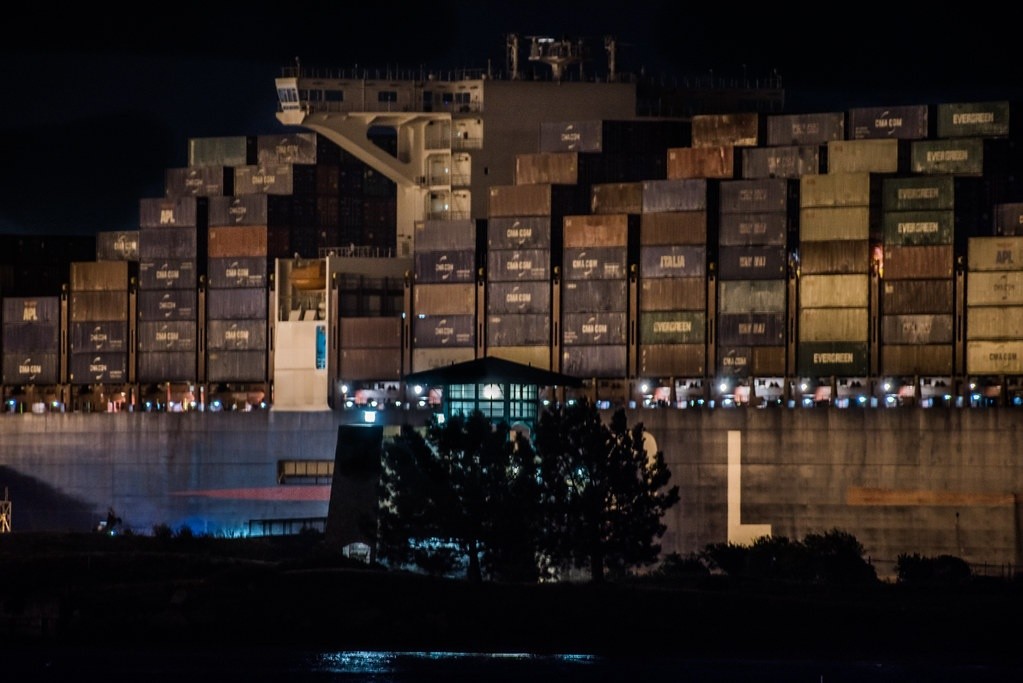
[0, 103, 1022, 383]
